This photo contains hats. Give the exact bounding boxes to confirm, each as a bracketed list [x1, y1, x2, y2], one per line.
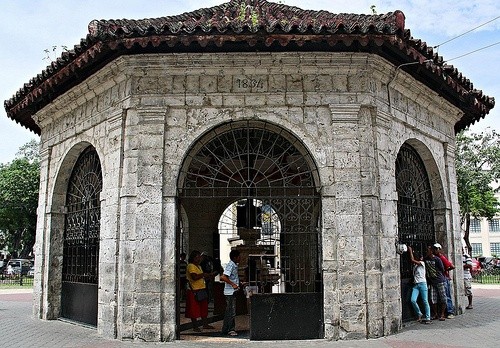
[433, 243, 442, 250]
[200, 252, 208, 256]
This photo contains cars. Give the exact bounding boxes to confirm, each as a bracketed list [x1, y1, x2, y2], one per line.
[26, 266, 34, 277]
[473, 256, 500, 274]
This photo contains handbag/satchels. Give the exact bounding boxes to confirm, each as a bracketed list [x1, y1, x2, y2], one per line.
[195, 289, 209, 302]
[231, 286, 248, 319]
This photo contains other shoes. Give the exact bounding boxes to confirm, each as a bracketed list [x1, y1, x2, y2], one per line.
[417, 313, 423, 322]
[203, 324, 215, 329]
[228, 331, 237, 336]
[466, 305, 473, 309]
[447, 314, 455, 318]
[425, 320, 431, 324]
[193, 327, 201, 332]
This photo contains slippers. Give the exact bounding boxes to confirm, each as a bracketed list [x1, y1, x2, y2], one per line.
[430, 316, 436, 320]
[439, 315, 445, 320]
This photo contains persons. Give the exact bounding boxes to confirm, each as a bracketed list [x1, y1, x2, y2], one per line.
[433, 243, 456, 319]
[188, 250, 215, 331]
[408, 246, 431, 324]
[424, 245, 447, 321]
[180, 253, 188, 302]
[462, 248, 473, 309]
[220, 250, 243, 336]
[201, 252, 212, 303]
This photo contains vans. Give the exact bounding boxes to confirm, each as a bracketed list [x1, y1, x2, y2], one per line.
[5, 259, 33, 277]
